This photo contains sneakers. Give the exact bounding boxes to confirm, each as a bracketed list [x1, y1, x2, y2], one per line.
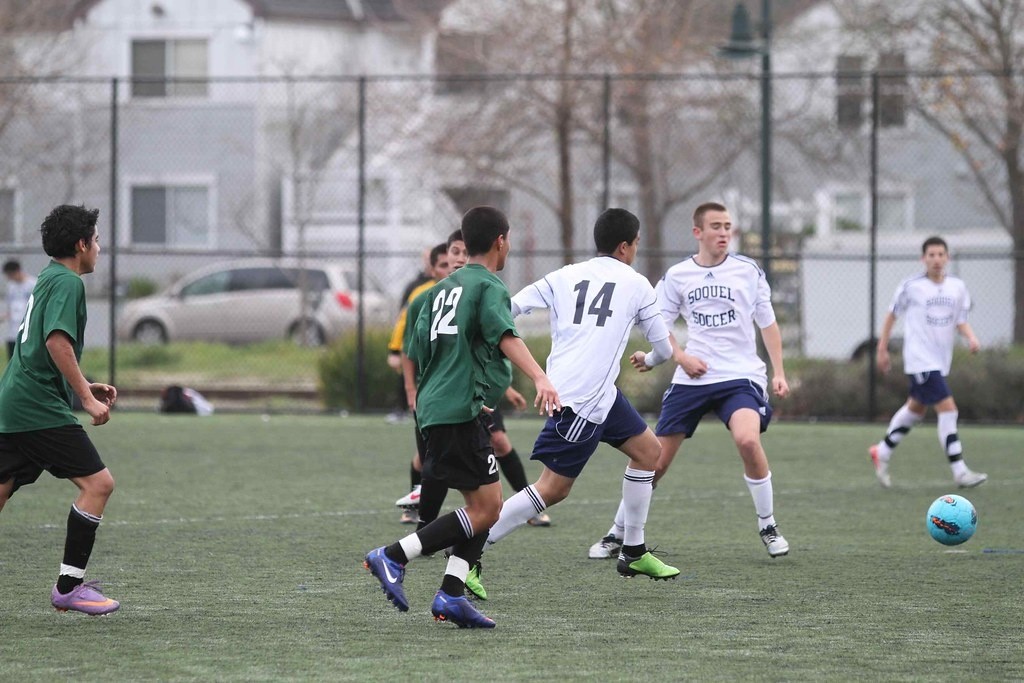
[432, 590, 496, 628]
[363, 545, 409, 612]
[616, 551, 681, 582]
[589, 534, 623, 558]
[529, 513, 552, 527]
[445, 547, 487, 600]
[51, 580, 119, 616]
[953, 470, 987, 488]
[869, 441, 892, 489]
[759, 522, 789, 557]
[395, 485, 422, 524]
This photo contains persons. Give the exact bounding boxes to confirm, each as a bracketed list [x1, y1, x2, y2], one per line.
[363, 205, 561, 628]
[868, 237, 988, 489]
[385, 229, 553, 526]
[0, 260, 39, 362]
[588, 204, 789, 560]
[0, 204, 120, 615]
[444, 207, 680, 601]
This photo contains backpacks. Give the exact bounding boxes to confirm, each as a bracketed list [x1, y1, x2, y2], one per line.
[161, 385, 213, 416]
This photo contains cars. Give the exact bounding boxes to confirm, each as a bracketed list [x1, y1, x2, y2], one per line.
[116, 257, 392, 348]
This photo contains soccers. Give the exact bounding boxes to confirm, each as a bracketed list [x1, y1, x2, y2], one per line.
[926, 493, 978, 546]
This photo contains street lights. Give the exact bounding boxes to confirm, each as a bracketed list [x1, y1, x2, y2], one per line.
[713, 0, 771, 293]
